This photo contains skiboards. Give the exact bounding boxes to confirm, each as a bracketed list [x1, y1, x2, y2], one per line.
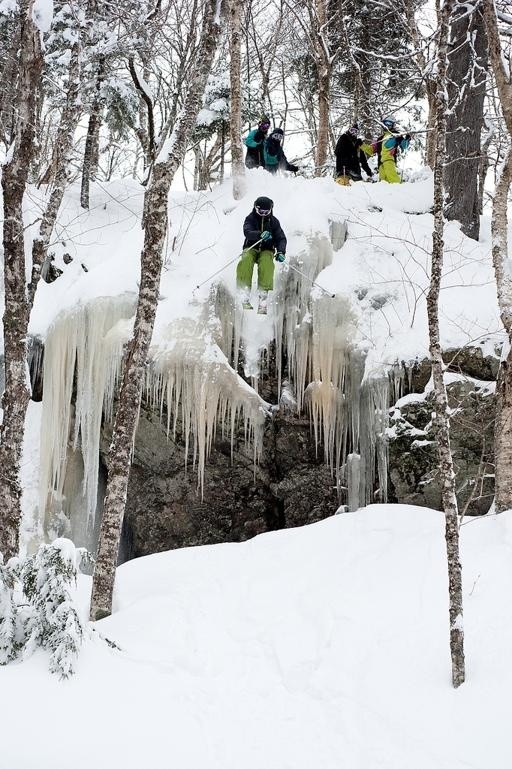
[242, 301, 267, 314]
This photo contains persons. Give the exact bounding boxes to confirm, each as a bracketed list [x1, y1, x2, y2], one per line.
[236, 197, 287, 314]
[334, 120, 411, 182]
[243, 114, 299, 172]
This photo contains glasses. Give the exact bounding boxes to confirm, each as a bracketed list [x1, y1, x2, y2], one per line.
[384, 121, 393, 126]
[349, 128, 360, 135]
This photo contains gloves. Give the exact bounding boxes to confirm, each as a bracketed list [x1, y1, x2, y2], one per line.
[260, 231, 273, 241]
[276, 252, 286, 262]
